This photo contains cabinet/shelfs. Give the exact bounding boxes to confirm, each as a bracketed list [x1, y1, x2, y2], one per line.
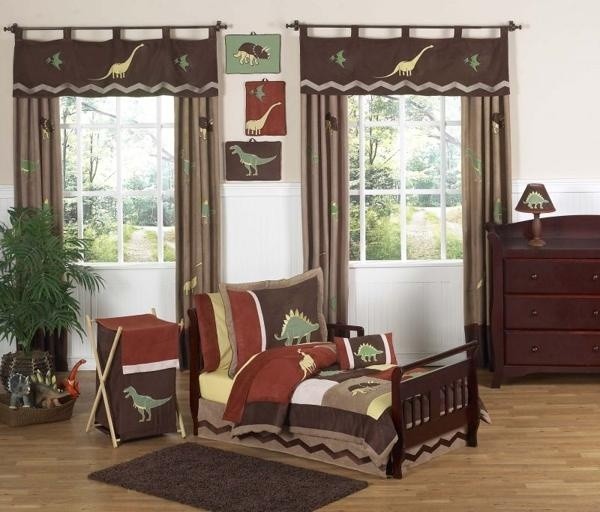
[484, 215, 600, 389]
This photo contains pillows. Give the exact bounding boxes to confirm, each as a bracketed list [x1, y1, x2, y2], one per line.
[333, 332, 399, 372]
[193, 266, 329, 379]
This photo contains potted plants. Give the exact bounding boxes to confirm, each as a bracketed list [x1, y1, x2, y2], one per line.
[0, 204, 109, 396]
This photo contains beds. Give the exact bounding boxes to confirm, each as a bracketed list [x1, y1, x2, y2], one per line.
[183, 309, 480, 480]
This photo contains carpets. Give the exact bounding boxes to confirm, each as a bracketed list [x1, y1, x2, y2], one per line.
[87, 442, 370, 512]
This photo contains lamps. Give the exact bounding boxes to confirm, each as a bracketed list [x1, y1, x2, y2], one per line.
[516, 183, 556, 247]
